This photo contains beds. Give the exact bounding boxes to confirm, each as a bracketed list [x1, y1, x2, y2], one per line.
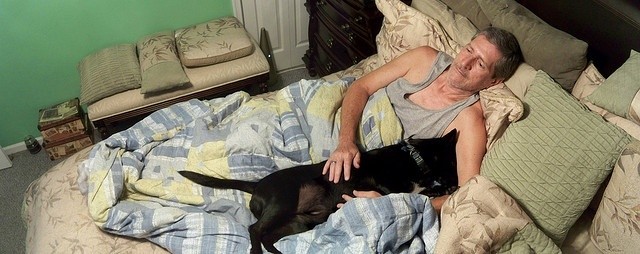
[21, 1, 640, 254]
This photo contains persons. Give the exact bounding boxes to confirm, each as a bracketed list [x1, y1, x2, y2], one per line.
[322, 27, 523, 216]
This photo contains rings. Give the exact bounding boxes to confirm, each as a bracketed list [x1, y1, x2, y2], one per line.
[330, 160, 336, 163]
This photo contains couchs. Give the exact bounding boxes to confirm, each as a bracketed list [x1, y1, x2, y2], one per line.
[86, 27, 272, 130]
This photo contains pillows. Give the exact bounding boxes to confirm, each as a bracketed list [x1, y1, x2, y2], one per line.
[411, 1, 538, 94]
[422, 0, 590, 94]
[369, 1, 524, 149]
[590, 142, 640, 252]
[77, 44, 140, 103]
[173, 13, 258, 68]
[585, 50, 640, 124]
[477, 68, 631, 249]
[137, 33, 190, 94]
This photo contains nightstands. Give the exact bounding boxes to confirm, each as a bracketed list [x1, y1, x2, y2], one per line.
[300, 1, 381, 79]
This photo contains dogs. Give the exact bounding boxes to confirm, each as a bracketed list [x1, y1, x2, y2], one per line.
[177, 127, 458, 254]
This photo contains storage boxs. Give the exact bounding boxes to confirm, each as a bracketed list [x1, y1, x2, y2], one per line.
[42, 112, 95, 160]
[38, 97, 85, 142]
